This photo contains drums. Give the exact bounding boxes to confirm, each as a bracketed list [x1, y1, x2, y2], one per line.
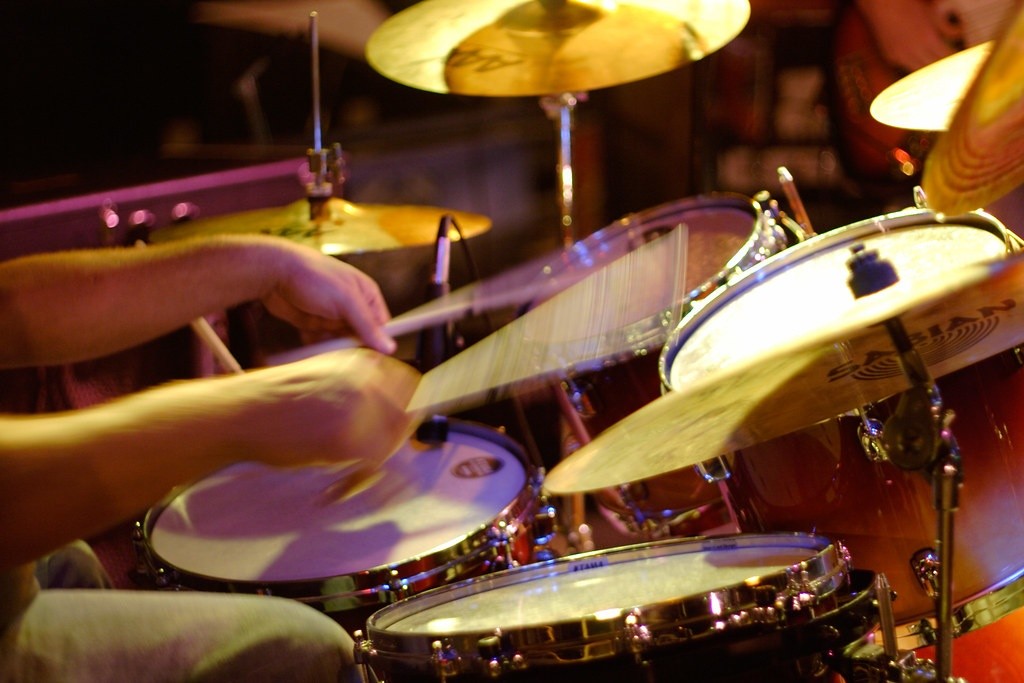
[138, 413, 549, 637]
[366, 0, 751, 97]
[527, 194, 796, 444]
[661, 205, 1024, 652]
[365, 529, 879, 683]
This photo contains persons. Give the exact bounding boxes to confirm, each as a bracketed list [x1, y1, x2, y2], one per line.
[0, 0, 419, 683]
[860, 0, 958, 72]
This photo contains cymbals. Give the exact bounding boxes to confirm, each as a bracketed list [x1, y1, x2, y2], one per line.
[922, 0, 1024, 216]
[150, 200, 490, 256]
[539, 252, 1024, 494]
[869, 43, 997, 136]
